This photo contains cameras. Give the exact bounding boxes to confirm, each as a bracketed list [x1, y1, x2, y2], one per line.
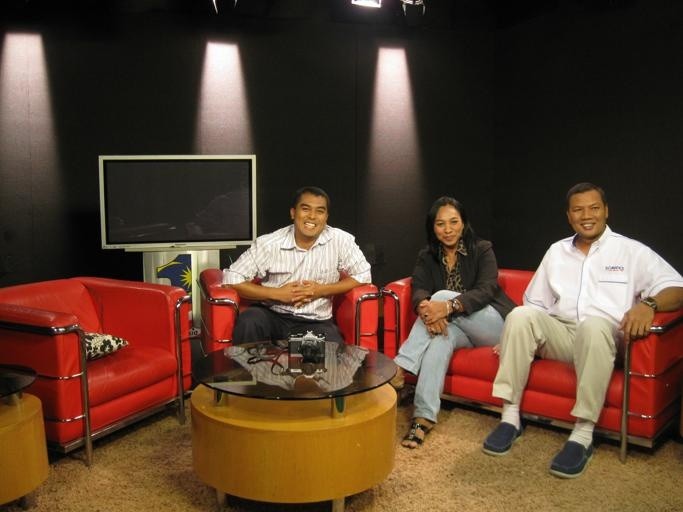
[289, 333, 327, 359]
[288, 358, 326, 379]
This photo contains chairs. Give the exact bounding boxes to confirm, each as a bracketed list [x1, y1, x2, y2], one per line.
[195, 265, 379, 362]
[1, 275, 195, 467]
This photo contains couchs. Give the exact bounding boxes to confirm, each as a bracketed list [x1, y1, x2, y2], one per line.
[381, 268, 683, 464]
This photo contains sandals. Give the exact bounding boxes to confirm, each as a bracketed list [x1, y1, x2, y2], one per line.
[402, 418, 434, 448]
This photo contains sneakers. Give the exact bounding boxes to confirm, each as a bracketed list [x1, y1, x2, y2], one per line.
[482, 422, 524, 457]
[549, 439, 593, 479]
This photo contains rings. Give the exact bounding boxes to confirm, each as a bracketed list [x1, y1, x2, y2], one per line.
[425, 314, 428, 317]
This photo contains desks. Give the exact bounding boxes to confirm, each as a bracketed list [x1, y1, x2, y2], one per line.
[0, 362, 50, 511]
[188, 340, 402, 511]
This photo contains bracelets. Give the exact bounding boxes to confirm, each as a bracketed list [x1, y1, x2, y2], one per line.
[445, 298, 451, 319]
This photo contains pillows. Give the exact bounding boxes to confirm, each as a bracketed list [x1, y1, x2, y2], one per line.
[85, 331, 129, 360]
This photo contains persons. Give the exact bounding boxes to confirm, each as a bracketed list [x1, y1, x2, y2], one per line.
[480, 180, 683, 480]
[226, 345, 374, 399]
[222, 183, 375, 347]
[384, 195, 519, 449]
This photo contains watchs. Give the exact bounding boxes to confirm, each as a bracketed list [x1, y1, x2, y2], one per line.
[451, 299, 461, 314]
[642, 295, 658, 310]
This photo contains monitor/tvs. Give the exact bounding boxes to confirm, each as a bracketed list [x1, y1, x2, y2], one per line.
[98, 153, 258, 253]
